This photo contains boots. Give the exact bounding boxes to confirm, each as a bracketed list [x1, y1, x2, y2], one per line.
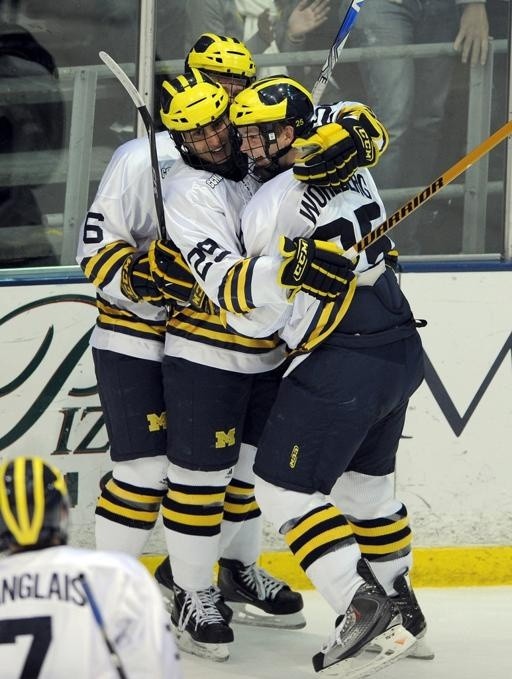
[154, 553, 304, 646]
[311, 558, 428, 673]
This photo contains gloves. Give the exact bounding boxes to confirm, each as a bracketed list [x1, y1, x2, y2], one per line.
[276, 234, 360, 304]
[120, 239, 206, 312]
[290, 106, 389, 189]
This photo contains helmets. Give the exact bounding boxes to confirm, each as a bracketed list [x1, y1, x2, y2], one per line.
[0, 455, 70, 552]
[159, 32, 315, 136]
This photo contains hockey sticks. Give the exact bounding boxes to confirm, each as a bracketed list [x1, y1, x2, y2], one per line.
[99, 51, 175, 322]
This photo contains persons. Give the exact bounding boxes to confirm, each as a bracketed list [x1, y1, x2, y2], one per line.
[0, 456, 180, 679]
